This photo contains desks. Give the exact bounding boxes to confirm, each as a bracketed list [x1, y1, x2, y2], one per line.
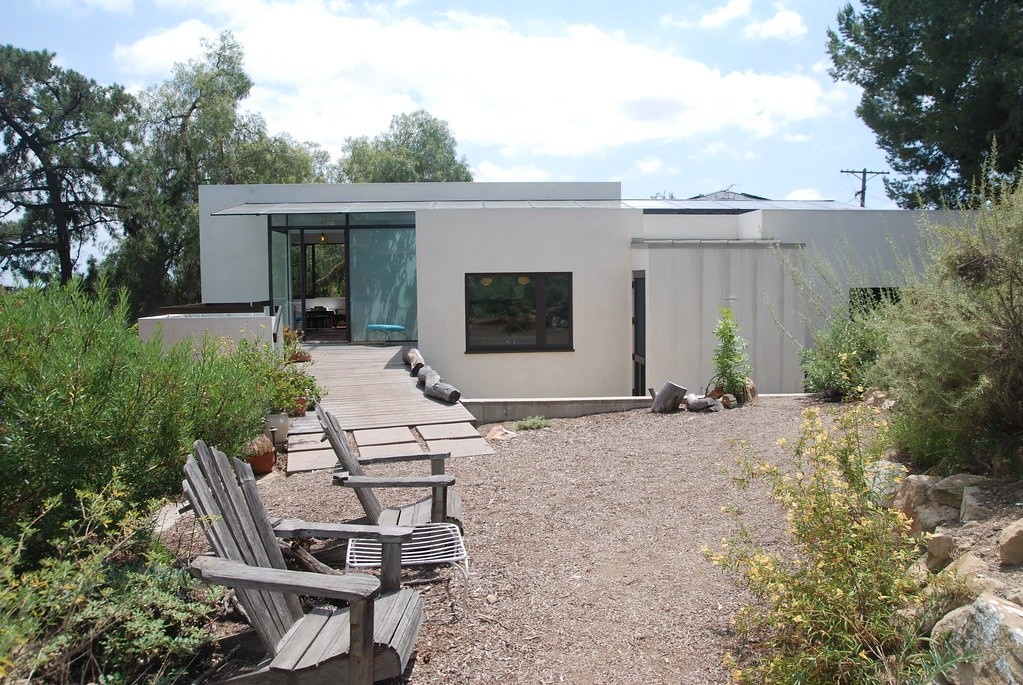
[305, 311, 334, 331]
[345, 522, 468, 619]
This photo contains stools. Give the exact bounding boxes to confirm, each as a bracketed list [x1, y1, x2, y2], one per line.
[366, 324, 410, 347]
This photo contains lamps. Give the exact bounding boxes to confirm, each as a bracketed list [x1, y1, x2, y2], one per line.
[318, 233, 328, 245]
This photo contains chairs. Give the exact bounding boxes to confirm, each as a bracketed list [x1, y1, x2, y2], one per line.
[178, 439, 426, 685]
[331, 308, 347, 328]
[312, 397, 465, 537]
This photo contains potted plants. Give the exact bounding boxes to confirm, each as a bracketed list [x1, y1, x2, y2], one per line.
[246, 434, 275, 475]
[272, 375, 329, 418]
[283, 325, 312, 361]
